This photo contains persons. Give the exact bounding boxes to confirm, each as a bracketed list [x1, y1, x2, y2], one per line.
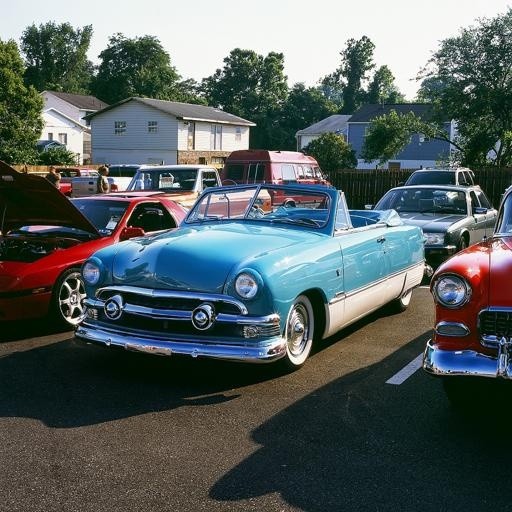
[45, 167, 61, 189]
[144, 174, 152, 189]
[97, 167, 109, 193]
